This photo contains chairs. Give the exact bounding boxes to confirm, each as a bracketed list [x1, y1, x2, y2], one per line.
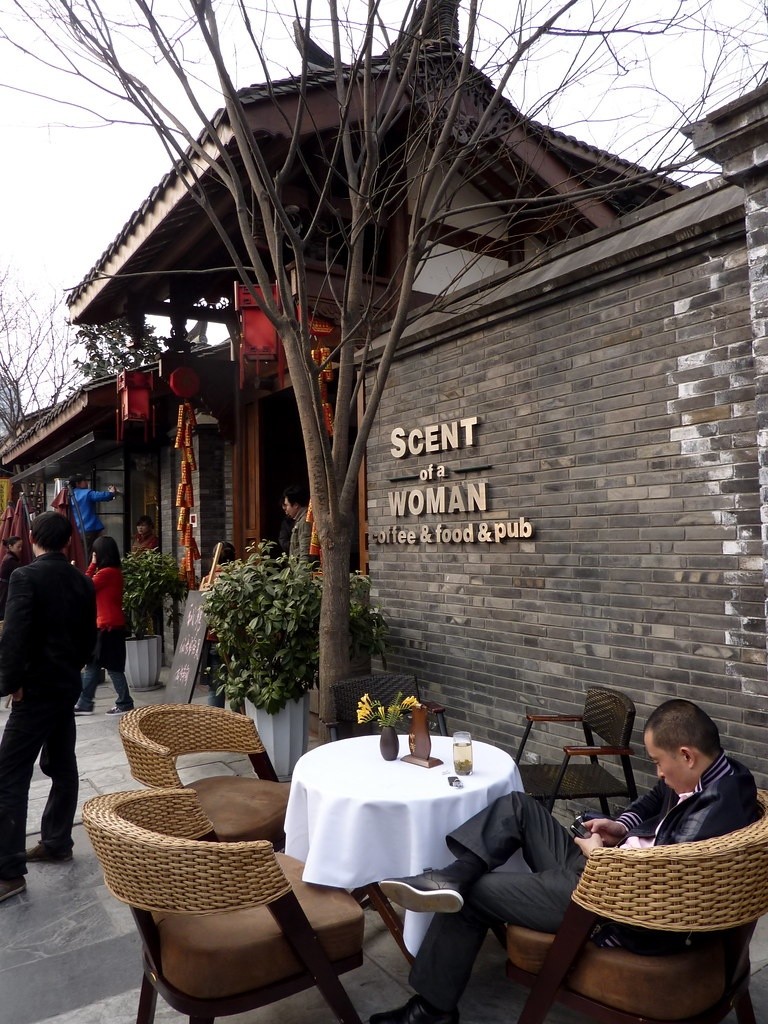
[318, 673, 448, 742]
[506, 787, 768, 1024]
[515, 685, 640, 813]
[80, 788, 365, 1024]
[118, 702, 290, 853]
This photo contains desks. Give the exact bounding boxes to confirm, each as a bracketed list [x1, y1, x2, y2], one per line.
[283, 734, 525, 970]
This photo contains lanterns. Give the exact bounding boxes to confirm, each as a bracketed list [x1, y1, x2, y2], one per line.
[114, 368, 158, 443]
[170, 367, 200, 400]
[233, 280, 286, 389]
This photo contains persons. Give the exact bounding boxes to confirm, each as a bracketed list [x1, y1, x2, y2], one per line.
[0, 509, 97, 901]
[367, 698, 758, 1024]
[68, 473, 117, 565]
[74, 536, 135, 716]
[280, 486, 318, 572]
[132, 516, 165, 653]
[0, 536, 23, 637]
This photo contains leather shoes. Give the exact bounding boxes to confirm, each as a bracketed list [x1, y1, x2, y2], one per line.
[378, 868, 464, 913]
[368, 994, 460, 1024]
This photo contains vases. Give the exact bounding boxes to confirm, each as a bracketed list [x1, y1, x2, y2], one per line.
[380, 727, 399, 761]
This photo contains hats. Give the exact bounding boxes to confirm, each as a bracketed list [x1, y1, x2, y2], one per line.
[66, 473, 85, 485]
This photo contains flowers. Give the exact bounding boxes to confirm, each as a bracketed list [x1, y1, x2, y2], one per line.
[356, 691, 421, 727]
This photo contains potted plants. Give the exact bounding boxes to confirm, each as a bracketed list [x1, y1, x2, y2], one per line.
[198, 538, 394, 783]
[124, 546, 189, 688]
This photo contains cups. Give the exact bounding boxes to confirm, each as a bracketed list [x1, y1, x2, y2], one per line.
[452, 731, 473, 776]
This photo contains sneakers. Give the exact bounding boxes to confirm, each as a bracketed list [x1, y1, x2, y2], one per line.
[0, 875, 26, 902]
[26, 840, 73, 862]
[74, 704, 94, 715]
[104, 706, 128, 715]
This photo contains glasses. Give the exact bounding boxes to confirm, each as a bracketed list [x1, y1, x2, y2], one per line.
[282, 503, 293, 510]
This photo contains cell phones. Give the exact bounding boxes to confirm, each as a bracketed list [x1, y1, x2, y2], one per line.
[448, 777, 460, 786]
[570, 820, 594, 840]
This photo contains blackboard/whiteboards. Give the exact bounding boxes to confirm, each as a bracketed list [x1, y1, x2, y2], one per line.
[163, 590, 212, 704]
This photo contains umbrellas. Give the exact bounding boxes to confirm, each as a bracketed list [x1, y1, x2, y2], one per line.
[0, 480, 87, 574]
